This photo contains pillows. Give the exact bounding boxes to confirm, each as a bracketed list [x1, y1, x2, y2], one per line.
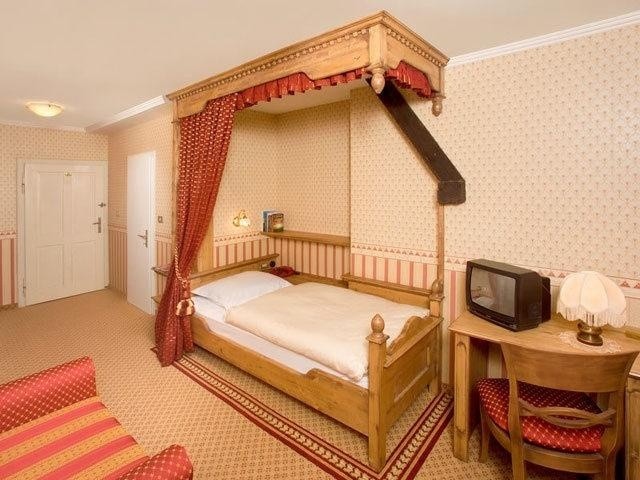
[189, 269, 294, 308]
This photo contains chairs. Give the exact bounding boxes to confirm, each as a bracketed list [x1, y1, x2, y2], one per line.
[475, 343, 640, 479]
[1, 357, 193, 480]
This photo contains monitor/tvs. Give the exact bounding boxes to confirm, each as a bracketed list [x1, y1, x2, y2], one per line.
[464, 258, 550, 331]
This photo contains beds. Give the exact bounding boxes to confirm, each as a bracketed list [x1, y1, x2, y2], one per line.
[185, 251, 446, 472]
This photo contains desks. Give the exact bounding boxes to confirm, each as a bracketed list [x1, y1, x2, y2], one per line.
[445, 309, 639, 480]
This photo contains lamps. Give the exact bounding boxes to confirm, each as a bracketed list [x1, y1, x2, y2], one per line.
[27, 102, 64, 118]
[554, 270, 628, 347]
[233, 211, 251, 227]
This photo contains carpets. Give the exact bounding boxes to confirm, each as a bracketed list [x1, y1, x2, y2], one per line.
[149, 340, 455, 480]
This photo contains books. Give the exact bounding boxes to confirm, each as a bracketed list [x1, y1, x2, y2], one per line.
[266, 211, 285, 234]
[261, 209, 274, 233]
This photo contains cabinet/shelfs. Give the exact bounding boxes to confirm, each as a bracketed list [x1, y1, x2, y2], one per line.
[149, 264, 171, 305]
